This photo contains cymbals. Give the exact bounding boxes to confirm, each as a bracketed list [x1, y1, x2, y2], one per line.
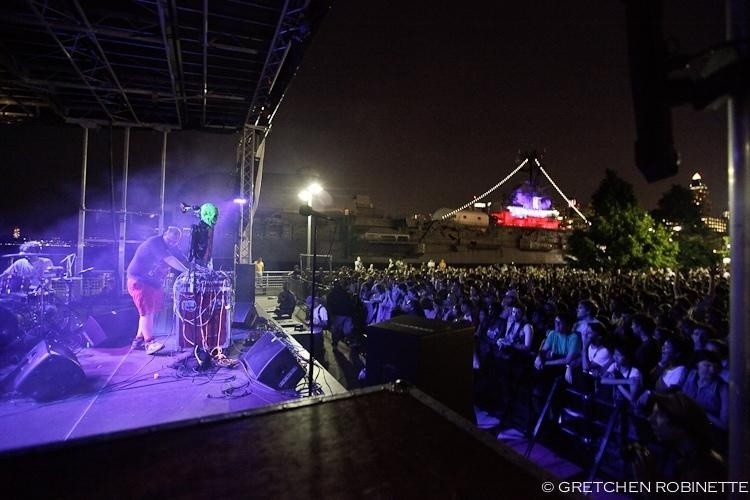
[2, 252, 50, 257]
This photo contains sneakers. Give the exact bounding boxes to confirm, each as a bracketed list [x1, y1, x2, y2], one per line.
[131, 339, 144, 350]
[145, 340, 165, 355]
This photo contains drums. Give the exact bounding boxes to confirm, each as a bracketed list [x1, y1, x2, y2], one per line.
[0, 274, 31, 298]
[0, 301, 33, 348]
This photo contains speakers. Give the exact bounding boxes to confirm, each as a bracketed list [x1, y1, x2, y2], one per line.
[232, 301, 259, 329]
[624, 0, 678, 183]
[366, 313, 477, 419]
[235, 263, 255, 303]
[243, 331, 306, 390]
[14, 340, 87, 401]
[83, 308, 139, 348]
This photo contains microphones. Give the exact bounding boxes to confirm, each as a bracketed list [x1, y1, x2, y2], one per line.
[0, 328, 10, 337]
[78, 267, 94, 275]
[36, 284, 42, 291]
[298, 205, 336, 221]
[59, 254, 74, 263]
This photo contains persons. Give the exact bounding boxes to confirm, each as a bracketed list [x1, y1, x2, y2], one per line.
[1, 241, 57, 345]
[186, 202, 219, 272]
[275, 282, 297, 320]
[254, 258, 264, 288]
[293, 257, 731, 500]
[126, 226, 190, 355]
